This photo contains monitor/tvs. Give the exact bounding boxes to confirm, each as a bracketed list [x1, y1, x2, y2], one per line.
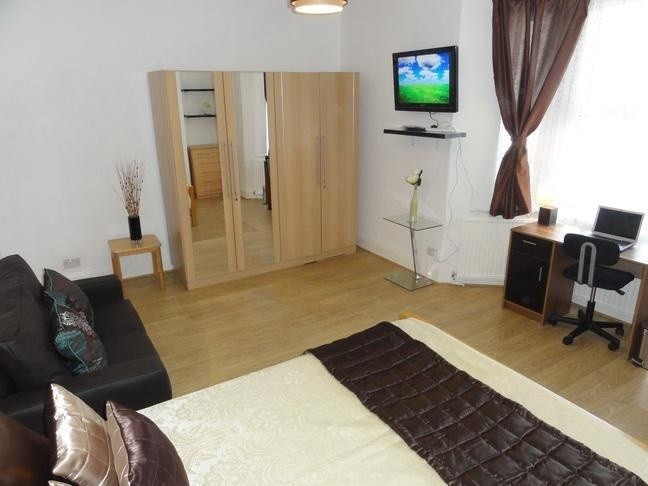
[392, 45, 459, 113]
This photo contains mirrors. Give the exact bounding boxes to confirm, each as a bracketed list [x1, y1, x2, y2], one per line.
[231, 70, 278, 271]
[177, 70, 231, 281]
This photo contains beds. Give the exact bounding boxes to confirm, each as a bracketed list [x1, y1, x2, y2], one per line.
[1, 317, 646, 486]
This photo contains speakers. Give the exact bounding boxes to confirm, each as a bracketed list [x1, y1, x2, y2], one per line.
[539, 205, 558, 226]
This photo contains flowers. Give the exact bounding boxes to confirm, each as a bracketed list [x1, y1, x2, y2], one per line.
[102, 150, 153, 218]
[406, 165, 424, 189]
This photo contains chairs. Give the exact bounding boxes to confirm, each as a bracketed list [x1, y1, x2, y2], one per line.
[548, 233, 635, 351]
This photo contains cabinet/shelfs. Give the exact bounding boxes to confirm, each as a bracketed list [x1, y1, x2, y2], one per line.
[180, 86, 216, 118]
[146, 69, 281, 293]
[278, 71, 361, 271]
[186, 143, 221, 199]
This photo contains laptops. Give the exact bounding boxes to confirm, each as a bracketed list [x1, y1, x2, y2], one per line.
[580, 206, 645, 252]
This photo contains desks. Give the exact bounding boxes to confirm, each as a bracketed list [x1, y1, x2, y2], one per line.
[109, 231, 163, 302]
[382, 213, 444, 292]
[501, 221, 646, 364]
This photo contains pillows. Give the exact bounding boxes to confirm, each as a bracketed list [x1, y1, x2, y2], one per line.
[104, 398, 191, 485]
[37, 380, 119, 485]
[41, 265, 97, 329]
[48, 298, 106, 377]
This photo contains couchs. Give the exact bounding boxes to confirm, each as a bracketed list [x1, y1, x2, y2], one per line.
[0, 253, 172, 437]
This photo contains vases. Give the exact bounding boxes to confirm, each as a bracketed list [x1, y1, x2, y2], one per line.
[127, 214, 145, 245]
[409, 186, 418, 225]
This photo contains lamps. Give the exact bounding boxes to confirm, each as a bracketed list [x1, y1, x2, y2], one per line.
[289, 0, 348, 16]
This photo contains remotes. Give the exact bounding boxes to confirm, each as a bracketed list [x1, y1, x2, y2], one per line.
[406, 126, 425, 131]
